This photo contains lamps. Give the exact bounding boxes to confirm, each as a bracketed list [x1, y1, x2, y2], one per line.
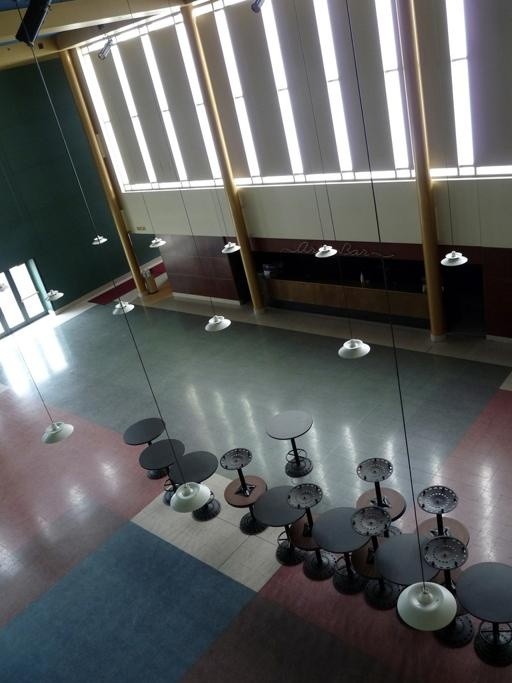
[196, 267, 233, 332]
[313, 227, 338, 259]
[220, 225, 243, 257]
[129, 173, 167, 250]
[337, 319, 371, 361]
[105, 271, 136, 317]
[69, 160, 110, 249]
[4, 341, 77, 446]
[439, 234, 466, 268]
[21, 213, 67, 304]
[395, 501, 458, 629]
[167, 434, 210, 514]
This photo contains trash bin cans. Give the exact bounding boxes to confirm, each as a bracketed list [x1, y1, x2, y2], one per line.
[143, 271, 158, 294]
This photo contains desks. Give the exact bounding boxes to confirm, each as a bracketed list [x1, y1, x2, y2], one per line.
[350, 508, 400, 610]
[378, 533, 438, 615]
[413, 486, 469, 547]
[287, 481, 336, 582]
[219, 447, 267, 535]
[356, 457, 407, 539]
[264, 410, 324, 475]
[255, 483, 303, 565]
[121, 418, 173, 479]
[140, 441, 185, 505]
[172, 451, 220, 521]
[456, 562, 512, 667]
[423, 536, 475, 647]
[312, 505, 361, 595]
